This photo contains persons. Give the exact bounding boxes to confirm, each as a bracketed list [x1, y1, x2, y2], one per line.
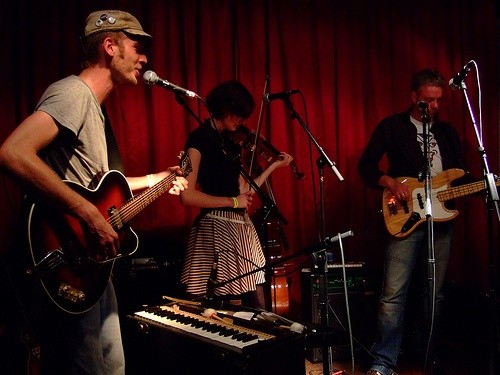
[358, 69, 497, 375]
[180, 80, 293, 310]
[0, 10, 188, 374]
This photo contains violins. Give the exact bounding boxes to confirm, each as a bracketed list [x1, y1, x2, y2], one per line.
[225, 124, 305, 180]
[243, 150, 291, 315]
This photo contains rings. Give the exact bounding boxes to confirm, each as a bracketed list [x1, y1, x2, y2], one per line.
[400, 193, 404, 195]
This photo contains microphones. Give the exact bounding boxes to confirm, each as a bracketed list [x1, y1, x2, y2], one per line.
[263, 89, 299, 104]
[417, 100, 427, 110]
[449, 62, 472, 90]
[143, 69, 196, 99]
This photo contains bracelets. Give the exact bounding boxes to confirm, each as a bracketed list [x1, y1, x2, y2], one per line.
[231, 197, 238, 208]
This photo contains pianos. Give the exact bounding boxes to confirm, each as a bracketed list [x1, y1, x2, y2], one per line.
[127, 304, 351, 375]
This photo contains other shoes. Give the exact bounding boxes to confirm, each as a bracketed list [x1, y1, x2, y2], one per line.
[366, 365, 391, 375]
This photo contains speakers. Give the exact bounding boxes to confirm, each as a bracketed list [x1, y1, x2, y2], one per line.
[302, 263, 385, 364]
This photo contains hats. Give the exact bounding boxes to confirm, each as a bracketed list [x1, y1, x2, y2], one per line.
[84, 10, 151, 38]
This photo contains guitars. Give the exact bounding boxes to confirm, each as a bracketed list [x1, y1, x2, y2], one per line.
[381, 168, 500, 238]
[28, 151, 193, 315]
